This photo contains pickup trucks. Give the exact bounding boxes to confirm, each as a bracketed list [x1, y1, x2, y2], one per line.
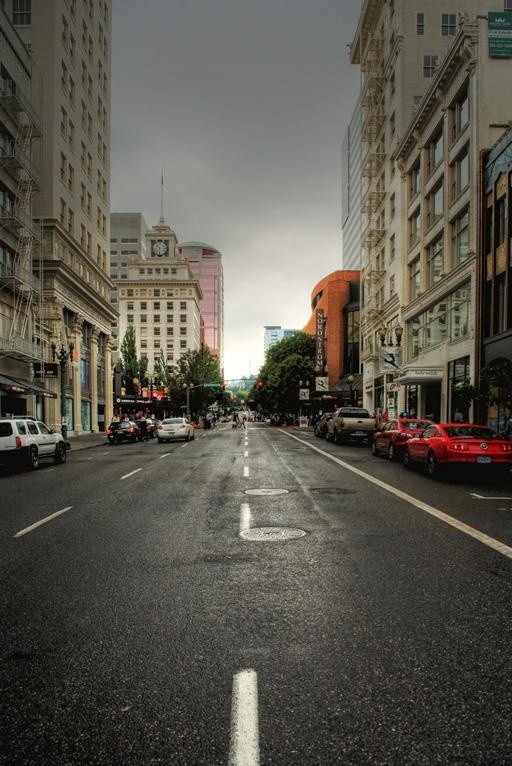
[325, 406, 379, 444]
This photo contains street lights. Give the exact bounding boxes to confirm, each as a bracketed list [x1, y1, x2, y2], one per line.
[345, 374, 355, 405]
[143, 376, 161, 401]
[47, 337, 76, 439]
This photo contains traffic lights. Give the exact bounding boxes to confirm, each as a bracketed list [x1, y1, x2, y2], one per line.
[258, 381, 263, 387]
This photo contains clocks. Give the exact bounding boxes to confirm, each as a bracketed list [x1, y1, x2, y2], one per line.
[153, 242, 167, 256]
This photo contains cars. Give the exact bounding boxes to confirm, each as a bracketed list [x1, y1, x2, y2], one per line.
[314, 411, 331, 438]
[369, 417, 436, 461]
[106, 417, 195, 442]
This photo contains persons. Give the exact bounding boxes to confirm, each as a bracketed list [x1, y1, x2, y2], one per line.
[111, 413, 120, 422]
[201, 410, 289, 429]
[410, 407, 416, 418]
[503, 406, 512, 439]
[139, 416, 148, 441]
[403, 411, 409, 418]
[120, 412, 129, 420]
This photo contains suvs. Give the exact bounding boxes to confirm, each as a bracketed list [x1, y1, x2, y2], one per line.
[1, 414, 68, 471]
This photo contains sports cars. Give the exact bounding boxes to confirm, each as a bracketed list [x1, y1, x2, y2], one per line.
[401, 420, 512, 482]
